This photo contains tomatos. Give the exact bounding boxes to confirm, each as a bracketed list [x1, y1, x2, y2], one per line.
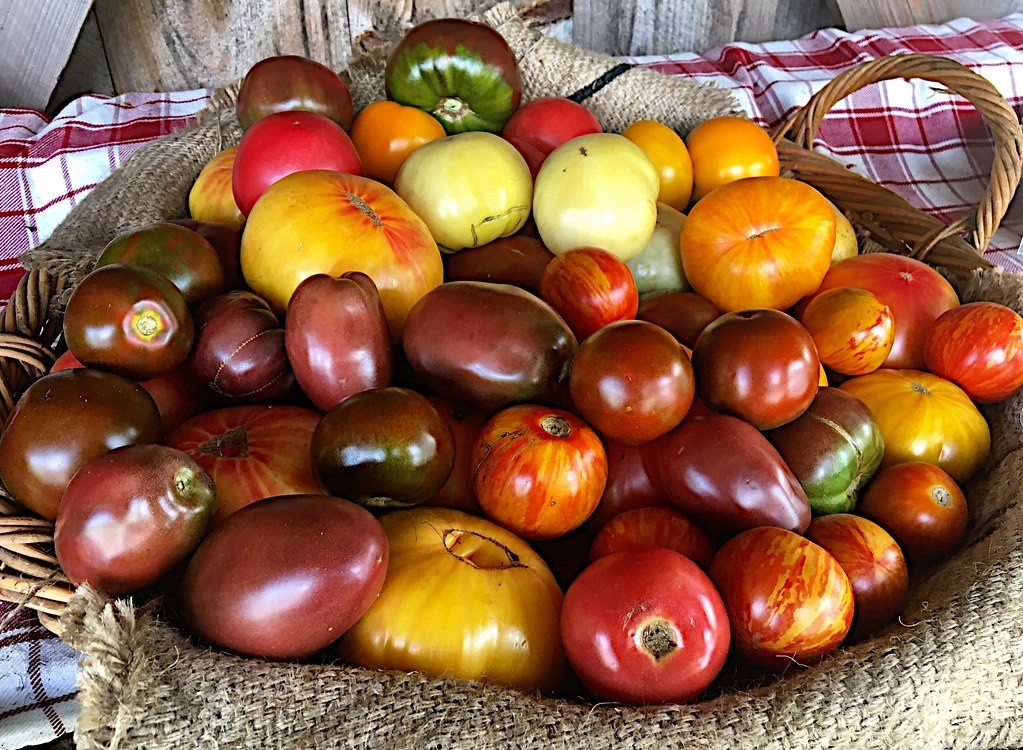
[0, 18, 1023, 704]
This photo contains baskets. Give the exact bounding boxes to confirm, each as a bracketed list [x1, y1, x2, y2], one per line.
[0, 55, 1023, 750]
